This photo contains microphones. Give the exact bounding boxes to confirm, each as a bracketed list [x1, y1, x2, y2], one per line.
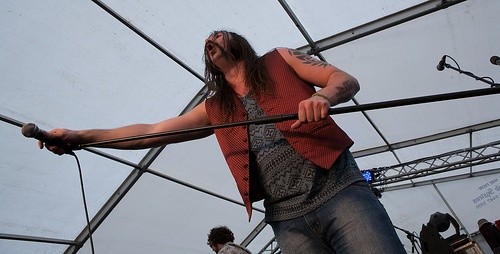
[22, 122, 75, 156]
[490, 56, 500, 65]
[437, 55, 446, 71]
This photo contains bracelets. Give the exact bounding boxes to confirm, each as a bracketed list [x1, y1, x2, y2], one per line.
[311, 93, 331, 104]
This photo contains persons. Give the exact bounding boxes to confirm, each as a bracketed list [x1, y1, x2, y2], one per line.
[206, 226, 251, 254]
[38, 28, 410, 254]
